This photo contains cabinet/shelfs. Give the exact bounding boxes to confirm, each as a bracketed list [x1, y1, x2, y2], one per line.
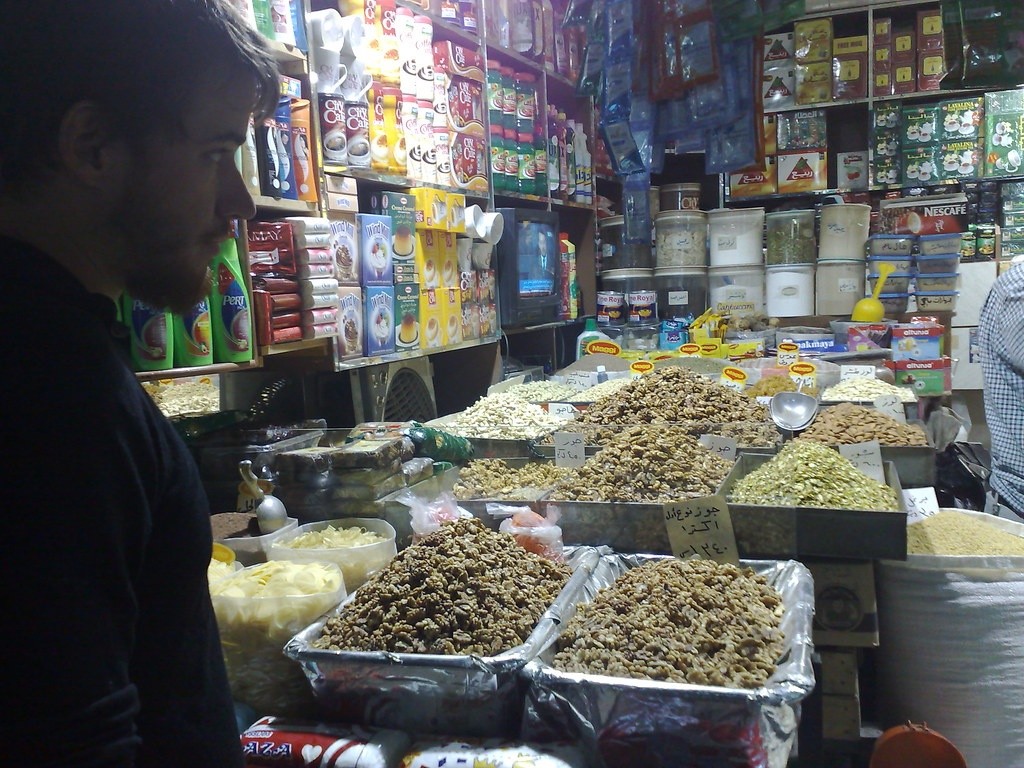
[723, 0, 1024, 201]
[127, 0, 622, 418]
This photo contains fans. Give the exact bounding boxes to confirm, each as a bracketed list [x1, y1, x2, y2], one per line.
[350, 356, 438, 424]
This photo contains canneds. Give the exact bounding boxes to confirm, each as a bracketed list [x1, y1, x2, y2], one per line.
[487, 59, 548, 196]
[596, 290, 626, 327]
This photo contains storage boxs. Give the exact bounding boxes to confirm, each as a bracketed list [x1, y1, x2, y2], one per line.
[283, 352, 936, 768]
[763, 16, 868, 108]
[839, 182, 1024, 261]
[881, 316, 952, 396]
[873, 9, 944, 98]
[950, 260, 1019, 390]
[279, 75, 302, 99]
[729, 109, 869, 197]
[873, 89, 1024, 185]
[323, 175, 466, 359]
[432, 40, 489, 192]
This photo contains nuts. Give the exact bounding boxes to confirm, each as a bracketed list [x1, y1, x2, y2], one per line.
[311, 517, 786, 690]
[432, 366, 781, 553]
[799, 377, 928, 446]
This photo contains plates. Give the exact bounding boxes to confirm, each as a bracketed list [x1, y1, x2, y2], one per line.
[877, 180, 896, 184]
[877, 151, 897, 156]
[907, 136, 931, 142]
[943, 165, 974, 173]
[877, 122, 896, 127]
[392, 234, 416, 260]
[395, 322, 420, 346]
[323, 147, 347, 160]
[944, 124, 975, 134]
[347, 156, 370, 165]
[907, 173, 931, 181]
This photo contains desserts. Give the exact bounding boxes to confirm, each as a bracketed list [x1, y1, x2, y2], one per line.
[628, 290, 659, 327]
[333, 225, 417, 344]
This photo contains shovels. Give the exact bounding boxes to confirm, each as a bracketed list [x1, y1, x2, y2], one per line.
[770, 377, 819, 431]
[851, 262, 896, 323]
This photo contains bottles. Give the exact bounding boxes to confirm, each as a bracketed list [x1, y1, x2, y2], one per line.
[396, 7, 452, 186]
[577, 319, 610, 383]
[318, 94, 347, 165]
[487, 59, 591, 205]
[558, 232, 577, 319]
[345, 100, 370, 165]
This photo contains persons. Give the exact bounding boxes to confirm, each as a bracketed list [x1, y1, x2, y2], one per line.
[0, 1, 280, 768]
[977, 260, 1024, 525]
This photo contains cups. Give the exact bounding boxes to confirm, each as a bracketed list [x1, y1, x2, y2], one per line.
[908, 169, 928, 179]
[992, 123, 1021, 172]
[877, 176, 895, 181]
[876, 147, 885, 153]
[347, 139, 370, 160]
[908, 130, 928, 139]
[946, 160, 969, 171]
[456, 203, 504, 272]
[947, 120, 970, 129]
[324, 132, 347, 154]
[311, 8, 372, 102]
